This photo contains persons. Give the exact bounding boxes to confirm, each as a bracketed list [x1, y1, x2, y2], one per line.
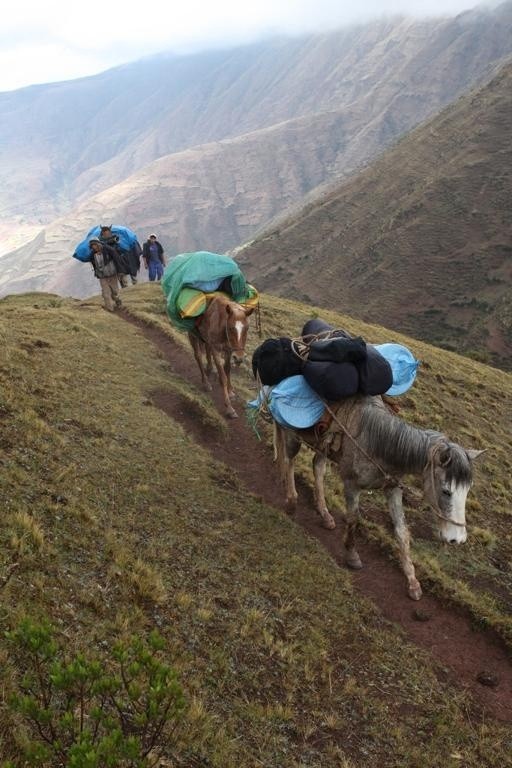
[86, 236, 123, 312]
[141, 232, 167, 280]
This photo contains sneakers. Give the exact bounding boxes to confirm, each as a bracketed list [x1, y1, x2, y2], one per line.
[115, 298, 123, 309]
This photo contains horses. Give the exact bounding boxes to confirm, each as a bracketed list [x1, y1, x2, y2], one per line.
[189, 288, 256, 421]
[99, 224, 115, 246]
[272, 388, 488, 602]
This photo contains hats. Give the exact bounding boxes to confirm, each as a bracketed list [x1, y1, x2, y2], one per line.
[88, 237, 103, 249]
[150, 233, 157, 239]
